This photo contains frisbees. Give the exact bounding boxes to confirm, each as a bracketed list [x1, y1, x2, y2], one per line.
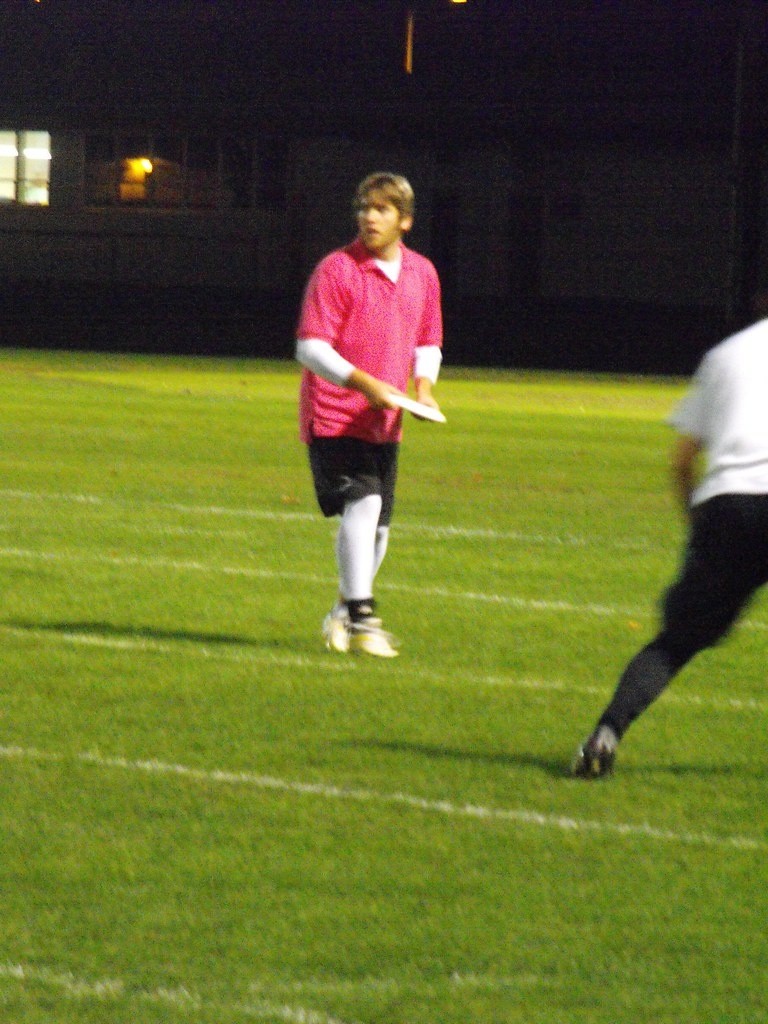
[384, 392, 446, 425]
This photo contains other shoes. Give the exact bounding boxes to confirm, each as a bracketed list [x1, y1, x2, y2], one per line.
[572, 746, 614, 778]
[323, 604, 351, 654]
[351, 618, 398, 658]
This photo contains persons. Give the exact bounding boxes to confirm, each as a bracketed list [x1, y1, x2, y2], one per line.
[295, 172, 443, 658]
[571, 317, 768, 777]
